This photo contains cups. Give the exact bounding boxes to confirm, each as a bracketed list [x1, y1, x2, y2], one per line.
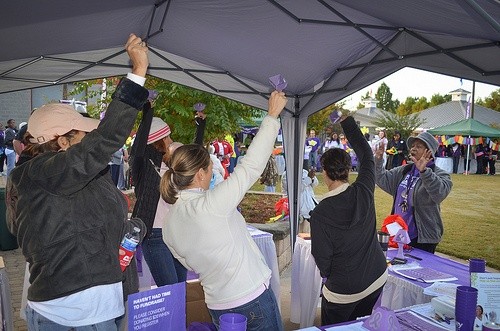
[329, 109, 343, 124]
[455, 286, 500, 331]
[469, 258, 486, 287]
[219, 313, 247, 331]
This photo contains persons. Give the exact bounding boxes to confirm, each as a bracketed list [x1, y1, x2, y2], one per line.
[373, 129, 452, 256]
[308, 112, 390, 328]
[106, 124, 362, 237]
[126, 91, 208, 331]
[152, 89, 288, 331]
[6, 32, 151, 331]
[365, 132, 500, 177]
[0, 114, 35, 185]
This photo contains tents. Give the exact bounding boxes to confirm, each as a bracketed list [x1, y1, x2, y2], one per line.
[394, 100, 500, 131]
[0, 0, 500, 331]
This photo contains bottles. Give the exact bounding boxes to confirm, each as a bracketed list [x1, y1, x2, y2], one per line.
[120, 226, 141, 251]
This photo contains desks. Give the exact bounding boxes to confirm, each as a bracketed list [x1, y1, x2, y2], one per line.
[136, 223, 282, 312]
[435, 157, 477, 174]
[290, 234, 500, 331]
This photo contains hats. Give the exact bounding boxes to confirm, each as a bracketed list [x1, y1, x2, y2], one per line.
[406, 132, 439, 160]
[147, 116, 172, 145]
[27, 103, 101, 144]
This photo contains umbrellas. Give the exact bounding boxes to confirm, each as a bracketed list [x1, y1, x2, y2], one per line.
[425, 119, 500, 176]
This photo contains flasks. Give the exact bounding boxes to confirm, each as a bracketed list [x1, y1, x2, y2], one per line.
[377, 231, 390, 258]
